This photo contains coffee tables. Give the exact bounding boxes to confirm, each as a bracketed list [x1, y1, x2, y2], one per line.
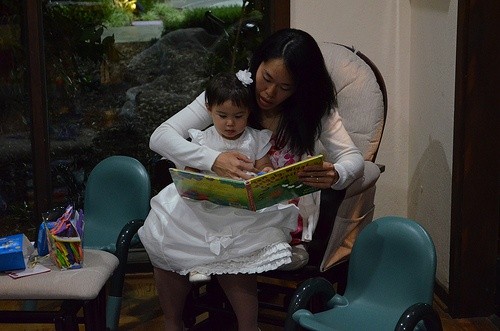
[0, 249, 120, 331]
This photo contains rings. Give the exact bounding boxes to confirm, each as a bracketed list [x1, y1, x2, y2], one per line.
[316, 177, 319, 184]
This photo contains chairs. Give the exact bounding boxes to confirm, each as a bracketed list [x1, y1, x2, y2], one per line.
[149, 42, 387, 331]
[284, 216, 444, 331]
[22, 155, 150, 331]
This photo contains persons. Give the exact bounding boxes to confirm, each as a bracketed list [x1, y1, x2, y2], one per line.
[137, 70, 298, 283]
[149, 27, 365, 331]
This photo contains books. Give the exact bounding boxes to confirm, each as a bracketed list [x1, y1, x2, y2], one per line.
[168, 151, 328, 212]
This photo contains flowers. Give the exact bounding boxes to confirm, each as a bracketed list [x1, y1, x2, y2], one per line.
[235, 70, 253, 86]
